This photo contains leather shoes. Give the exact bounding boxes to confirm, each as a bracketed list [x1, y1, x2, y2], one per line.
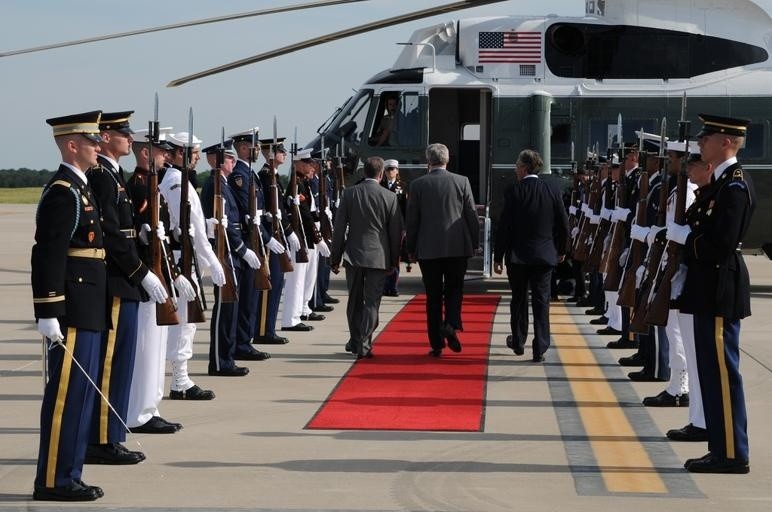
[667, 424, 709, 442]
[31, 479, 102, 501]
[643, 391, 689, 408]
[441, 323, 460, 352]
[430, 349, 442, 357]
[84, 444, 143, 464]
[506, 335, 524, 355]
[344, 341, 371, 360]
[282, 294, 339, 332]
[567, 294, 620, 335]
[684, 451, 750, 473]
[210, 333, 287, 375]
[128, 414, 181, 434]
[532, 353, 545, 363]
[171, 386, 214, 400]
[608, 338, 668, 383]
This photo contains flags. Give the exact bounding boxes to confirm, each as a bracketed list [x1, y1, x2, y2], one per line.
[477, 31, 542, 64]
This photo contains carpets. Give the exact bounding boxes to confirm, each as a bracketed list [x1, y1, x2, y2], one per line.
[294, 291, 505, 432]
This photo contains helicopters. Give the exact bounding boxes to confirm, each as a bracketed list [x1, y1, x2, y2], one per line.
[1, 0, 771, 277]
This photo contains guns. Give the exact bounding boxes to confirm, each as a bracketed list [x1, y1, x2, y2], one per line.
[568, 90, 690, 336]
[181, 107, 207, 325]
[248, 128, 271, 290]
[153, 94, 161, 145]
[147, 120, 179, 325]
[272, 116, 294, 273]
[291, 132, 346, 263]
[213, 127, 240, 303]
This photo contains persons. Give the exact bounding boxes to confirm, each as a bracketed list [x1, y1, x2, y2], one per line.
[400, 141, 483, 359]
[368, 93, 412, 150]
[379, 157, 408, 298]
[324, 156, 403, 360]
[556, 114, 753, 472]
[490, 148, 570, 362]
[32, 110, 346, 500]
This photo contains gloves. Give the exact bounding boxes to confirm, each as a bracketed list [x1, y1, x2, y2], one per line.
[135, 223, 197, 304]
[571, 227, 579, 238]
[209, 263, 225, 286]
[206, 217, 226, 237]
[318, 239, 331, 258]
[325, 207, 333, 220]
[265, 236, 284, 254]
[38, 317, 63, 341]
[244, 214, 262, 226]
[569, 201, 630, 224]
[619, 249, 629, 266]
[242, 247, 260, 270]
[288, 195, 301, 209]
[670, 265, 687, 300]
[288, 232, 300, 252]
[630, 218, 693, 245]
[636, 266, 648, 287]
[265, 207, 281, 222]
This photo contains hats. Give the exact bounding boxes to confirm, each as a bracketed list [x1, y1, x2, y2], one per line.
[587, 144, 636, 169]
[135, 127, 202, 150]
[46, 109, 105, 145]
[637, 129, 701, 156]
[383, 159, 398, 169]
[99, 110, 134, 136]
[698, 113, 750, 139]
[294, 146, 331, 161]
[205, 128, 286, 156]
